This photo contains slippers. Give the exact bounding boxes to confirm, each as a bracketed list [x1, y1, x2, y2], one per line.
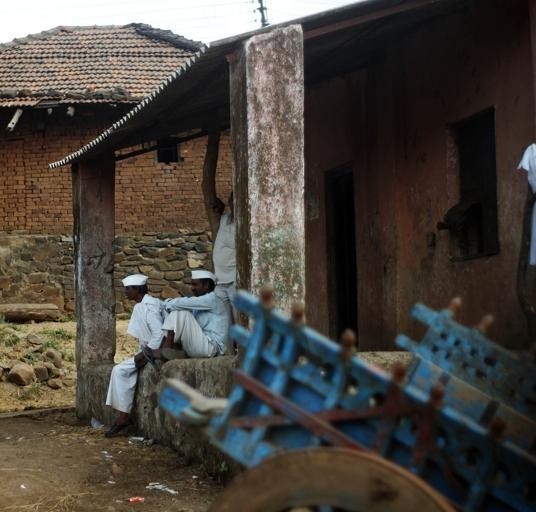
[139, 343, 159, 374]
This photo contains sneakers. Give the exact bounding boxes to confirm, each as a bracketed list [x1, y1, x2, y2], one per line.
[160, 346, 186, 362]
[106, 416, 134, 438]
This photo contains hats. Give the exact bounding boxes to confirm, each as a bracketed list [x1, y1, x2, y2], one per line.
[191, 270, 216, 283]
[122, 273, 148, 287]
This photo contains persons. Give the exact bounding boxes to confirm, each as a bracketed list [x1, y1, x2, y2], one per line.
[104, 273, 166, 437]
[141, 269, 229, 366]
[211, 190, 237, 354]
